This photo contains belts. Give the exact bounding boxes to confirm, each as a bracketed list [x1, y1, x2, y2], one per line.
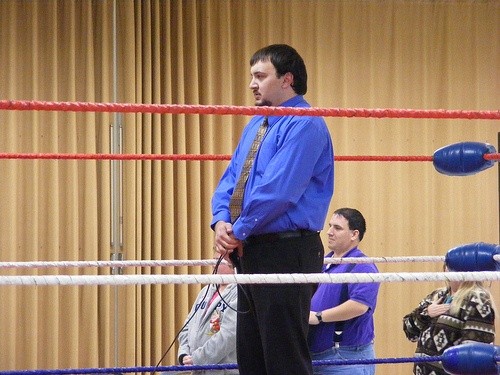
[244, 228, 318, 245]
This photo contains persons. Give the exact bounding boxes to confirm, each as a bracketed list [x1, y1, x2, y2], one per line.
[403, 263, 496, 375]
[176, 41, 380, 375]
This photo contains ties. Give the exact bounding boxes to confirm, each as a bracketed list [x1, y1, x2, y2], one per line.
[230, 116, 268, 223]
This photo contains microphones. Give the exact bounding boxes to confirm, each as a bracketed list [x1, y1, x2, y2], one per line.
[229, 251, 237, 270]
